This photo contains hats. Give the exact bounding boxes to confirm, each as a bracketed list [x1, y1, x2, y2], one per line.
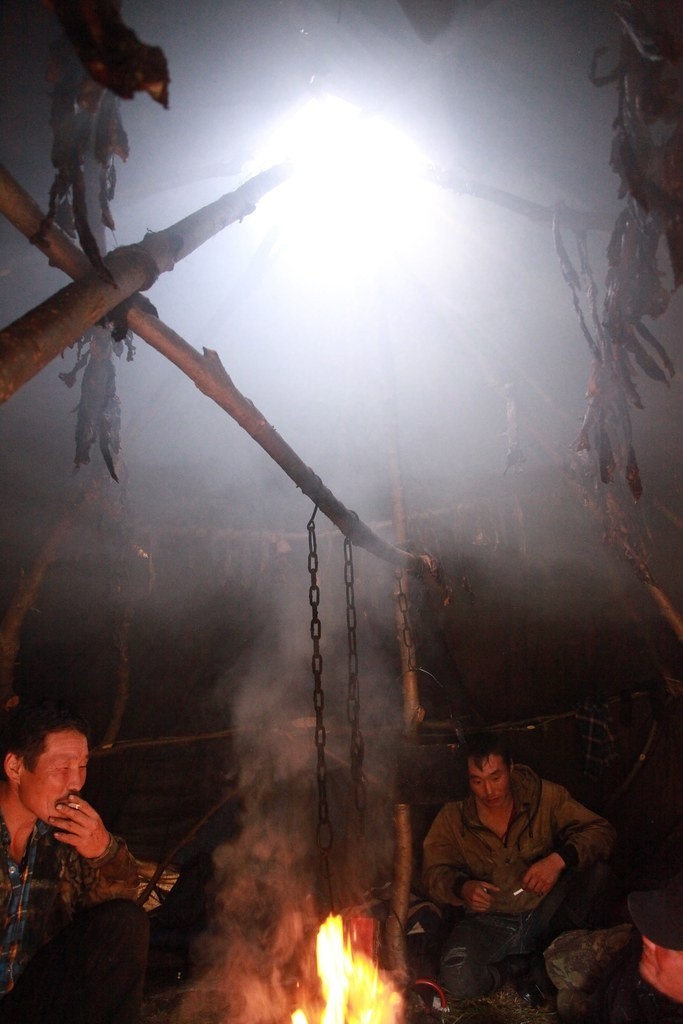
[627, 873, 682, 951]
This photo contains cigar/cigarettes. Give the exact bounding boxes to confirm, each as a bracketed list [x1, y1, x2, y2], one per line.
[64, 802, 81, 810]
[512, 888, 524, 896]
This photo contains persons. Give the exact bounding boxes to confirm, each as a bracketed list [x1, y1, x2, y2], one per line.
[543, 873, 683, 1024]
[0, 696, 152, 1024]
[420, 736, 619, 1011]
